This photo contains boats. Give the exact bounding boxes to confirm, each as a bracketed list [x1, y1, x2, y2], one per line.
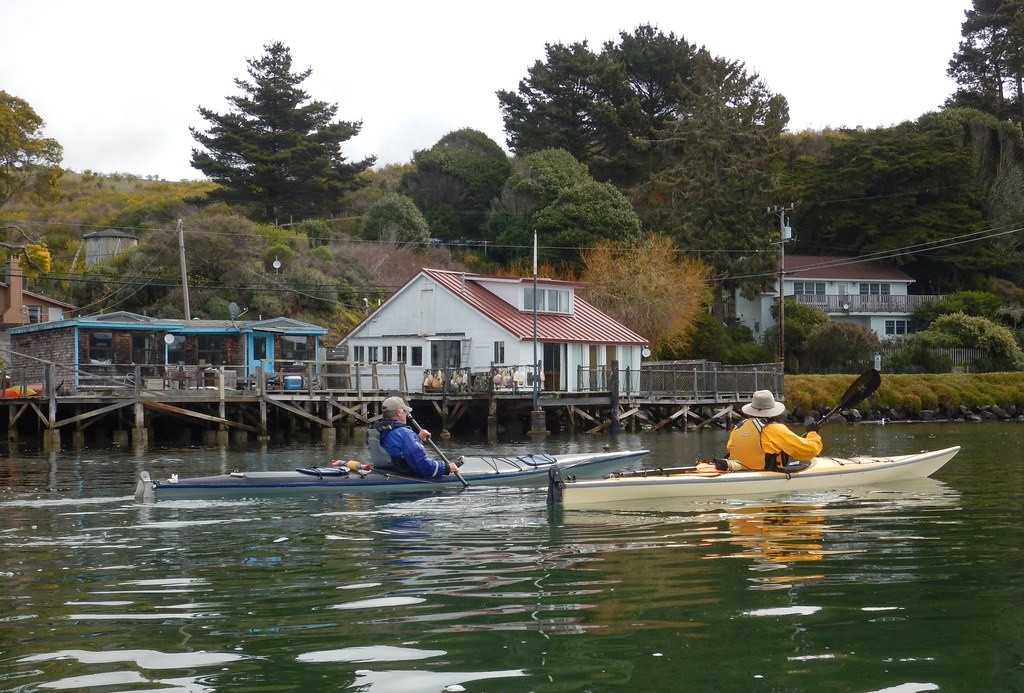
[546, 445, 961, 511]
[134, 449, 652, 500]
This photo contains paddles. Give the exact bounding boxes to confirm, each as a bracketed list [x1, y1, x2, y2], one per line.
[800, 368, 882, 438]
[386, 390, 470, 488]
[601, 460, 732, 479]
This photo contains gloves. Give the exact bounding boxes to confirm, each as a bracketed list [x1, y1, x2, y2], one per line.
[806, 422, 820, 433]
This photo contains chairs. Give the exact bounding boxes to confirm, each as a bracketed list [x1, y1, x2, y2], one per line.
[163, 364, 181, 390]
[182, 365, 205, 390]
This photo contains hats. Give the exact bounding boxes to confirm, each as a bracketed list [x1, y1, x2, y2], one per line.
[381, 396, 412, 412]
[742, 389, 785, 417]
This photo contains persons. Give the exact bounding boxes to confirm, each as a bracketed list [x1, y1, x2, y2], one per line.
[727, 390, 823, 470]
[365, 396, 458, 479]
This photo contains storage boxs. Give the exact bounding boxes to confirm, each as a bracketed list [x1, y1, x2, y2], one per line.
[284, 376, 302, 389]
